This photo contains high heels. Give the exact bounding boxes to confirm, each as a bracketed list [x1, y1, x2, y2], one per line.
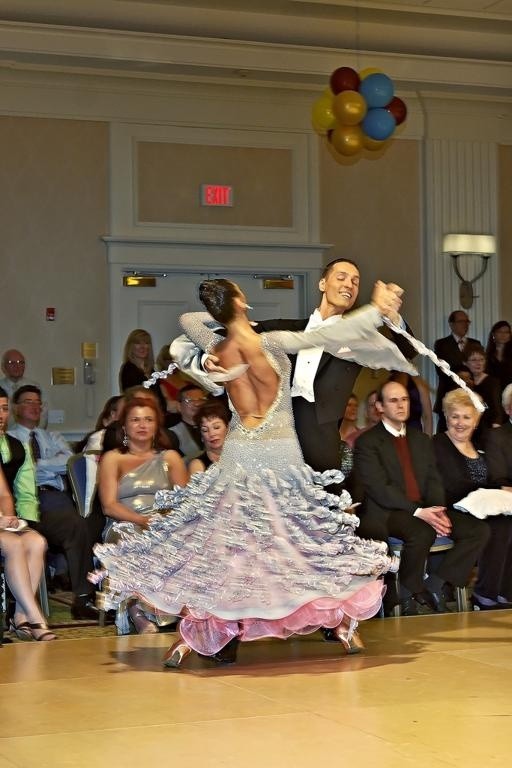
[471, 593, 506, 611]
[496, 599, 512, 609]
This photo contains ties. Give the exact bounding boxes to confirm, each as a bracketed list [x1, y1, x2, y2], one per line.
[30, 431, 41, 463]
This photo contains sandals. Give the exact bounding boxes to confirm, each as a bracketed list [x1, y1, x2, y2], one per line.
[162, 639, 192, 667]
[130, 611, 160, 634]
[335, 621, 364, 654]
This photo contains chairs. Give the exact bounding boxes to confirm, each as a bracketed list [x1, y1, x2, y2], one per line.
[67, 450, 132, 634]
[390, 535, 471, 618]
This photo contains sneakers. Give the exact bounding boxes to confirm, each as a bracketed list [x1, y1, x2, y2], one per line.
[37, 485, 58, 491]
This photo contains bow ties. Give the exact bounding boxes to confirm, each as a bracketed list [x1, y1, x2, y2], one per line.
[310, 315, 340, 328]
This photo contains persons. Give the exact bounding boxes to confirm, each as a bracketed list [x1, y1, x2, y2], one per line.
[0, 349, 48, 431]
[121, 329, 183, 453]
[343, 389, 384, 447]
[433, 388, 512, 611]
[352, 382, 491, 617]
[104, 387, 182, 456]
[432, 310, 482, 434]
[461, 345, 503, 455]
[0, 388, 40, 529]
[483, 382, 512, 493]
[189, 405, 231, 479]
[89, 276, 402, 669]
[485, 321, 512, 395]
[451, 365, 475, 386]
[98, 398, 189, 634]
[0, 465, 56, 641]
[84, 395, 126, 451]
[6, 385, 115, 619]
[339, 393, 361, 440]
[166, 385, 208, 463]
[156, 343, 188, 414]
[384, 369, 433, 437]
[199, 260, 415, 666]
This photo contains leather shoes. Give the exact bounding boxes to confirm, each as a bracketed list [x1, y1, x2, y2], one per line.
[424, 577, 444, 612]
[72, 599, 114, 620]
[198, 641, 238, 664]
[323, 628, 334, 640]
[413, 593, 432, 614]
[6, 612, 15, 626]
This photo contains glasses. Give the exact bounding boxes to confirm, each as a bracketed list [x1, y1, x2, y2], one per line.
[466, 359, 484, 363]
[453, 320, 471, 325]
[182, 397, 208, 403]
[5, 360, 26, 366]
[16, 399, 42, 406]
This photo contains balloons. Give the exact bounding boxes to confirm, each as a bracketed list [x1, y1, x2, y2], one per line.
[314, 66, 408, 164]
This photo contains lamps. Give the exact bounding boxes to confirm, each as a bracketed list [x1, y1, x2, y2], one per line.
[443, 232, 496, 310]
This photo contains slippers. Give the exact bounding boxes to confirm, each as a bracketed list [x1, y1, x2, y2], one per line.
[9, 618, 33, 641]
[29, 623, 59, 641]
